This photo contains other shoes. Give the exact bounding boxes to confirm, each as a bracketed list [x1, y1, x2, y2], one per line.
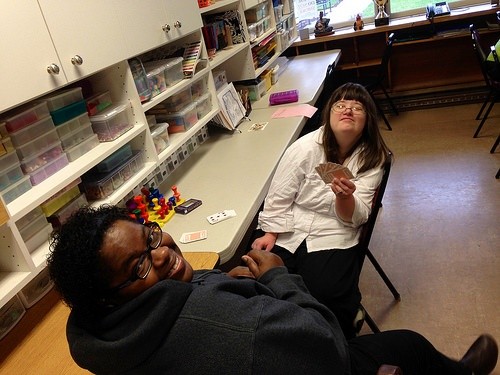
[461, 332, 499, 375]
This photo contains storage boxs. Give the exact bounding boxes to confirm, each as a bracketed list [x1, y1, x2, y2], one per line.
[0, 0, 295, 339]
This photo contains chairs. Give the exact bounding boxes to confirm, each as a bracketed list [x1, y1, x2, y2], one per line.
[333, 32, 406, 135]
[470, 32, 500, 138]
[353, 141, 402, 333]
[489, 50, 500, 181]
[468, 23, 500, 120]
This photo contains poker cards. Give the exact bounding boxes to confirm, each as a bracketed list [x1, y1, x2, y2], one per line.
[238, 274, 253, 278]
[315, 162, 356, 184]
[206, 209, 237, 225]
[179, 230, 208, 244]
[248, 121, 270, 131]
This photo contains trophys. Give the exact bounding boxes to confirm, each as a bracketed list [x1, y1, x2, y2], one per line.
[373, 0, 389, 27]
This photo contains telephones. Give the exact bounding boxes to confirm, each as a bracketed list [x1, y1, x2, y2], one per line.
[426, 1, 451, 20]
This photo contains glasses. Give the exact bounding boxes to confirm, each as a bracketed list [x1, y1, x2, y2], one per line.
[332, 102, 367, 115]
[109, 222, 163, 290]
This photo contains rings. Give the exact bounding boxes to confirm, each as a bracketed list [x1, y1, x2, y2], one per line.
[340, 190, 344, 193]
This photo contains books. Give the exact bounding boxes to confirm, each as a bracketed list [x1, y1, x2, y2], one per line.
[202, 21, 226, 51]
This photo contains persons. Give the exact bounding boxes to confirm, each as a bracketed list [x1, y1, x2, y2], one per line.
[251, 83, 394, 340]
[314, 11, 333, 34]
[46, 204, 498, 375]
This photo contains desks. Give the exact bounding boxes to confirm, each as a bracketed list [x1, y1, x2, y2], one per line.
[249, 48, 342, 106]
[155, 103, 314, 266]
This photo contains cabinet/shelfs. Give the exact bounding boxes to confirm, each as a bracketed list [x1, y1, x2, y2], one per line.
[0, 0, 205, 115]
[0, 29, 223, 272]
[290, 1, 500, 98]
[198, 0, 249, 71]
[211, 0, 298, 82]
